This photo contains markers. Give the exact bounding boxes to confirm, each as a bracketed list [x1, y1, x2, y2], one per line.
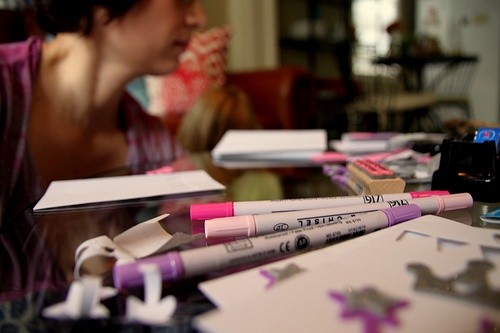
[203, 192, 474, 239]
[111, 204, 421, 292]
[190, 190, 450, 222]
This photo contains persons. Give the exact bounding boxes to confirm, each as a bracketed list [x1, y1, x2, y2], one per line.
[0, 0, 207, 304]
